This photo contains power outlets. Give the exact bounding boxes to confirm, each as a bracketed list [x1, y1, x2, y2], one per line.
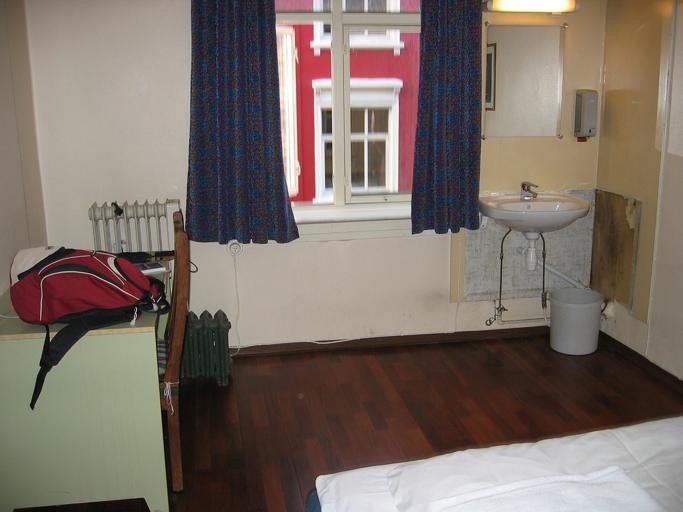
[227, 239, 243, 256]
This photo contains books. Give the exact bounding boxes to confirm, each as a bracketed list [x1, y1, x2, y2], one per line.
[136, 262, 165, 274]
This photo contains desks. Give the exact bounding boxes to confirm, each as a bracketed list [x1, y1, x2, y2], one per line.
[0, 260, 172, 512]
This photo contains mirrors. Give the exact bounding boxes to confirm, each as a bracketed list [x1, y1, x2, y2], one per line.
[482, 21, 568, 139]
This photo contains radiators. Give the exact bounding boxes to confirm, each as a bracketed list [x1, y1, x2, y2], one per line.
[89, 198, 181, 254]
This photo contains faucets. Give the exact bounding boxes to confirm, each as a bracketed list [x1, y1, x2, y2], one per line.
[519, 183, 538, 200]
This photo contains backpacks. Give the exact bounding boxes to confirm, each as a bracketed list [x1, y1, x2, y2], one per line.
[9, 247, 170, 410]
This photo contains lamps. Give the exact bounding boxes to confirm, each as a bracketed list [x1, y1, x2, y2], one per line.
[486, 0, 581, 15]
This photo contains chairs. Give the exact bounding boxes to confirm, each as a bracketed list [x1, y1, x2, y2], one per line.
[157, 211, 190, 493]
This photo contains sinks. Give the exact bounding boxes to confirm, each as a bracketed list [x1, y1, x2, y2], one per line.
[479, 196, 590, 232]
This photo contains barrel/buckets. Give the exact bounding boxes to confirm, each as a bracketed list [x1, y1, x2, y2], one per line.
[549, 288, 604, 355]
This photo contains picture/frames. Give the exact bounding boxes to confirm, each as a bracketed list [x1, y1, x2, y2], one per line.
[485, 43, 496, 111]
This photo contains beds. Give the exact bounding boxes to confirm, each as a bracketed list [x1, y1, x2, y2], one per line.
[306, 416, 683, 512]
